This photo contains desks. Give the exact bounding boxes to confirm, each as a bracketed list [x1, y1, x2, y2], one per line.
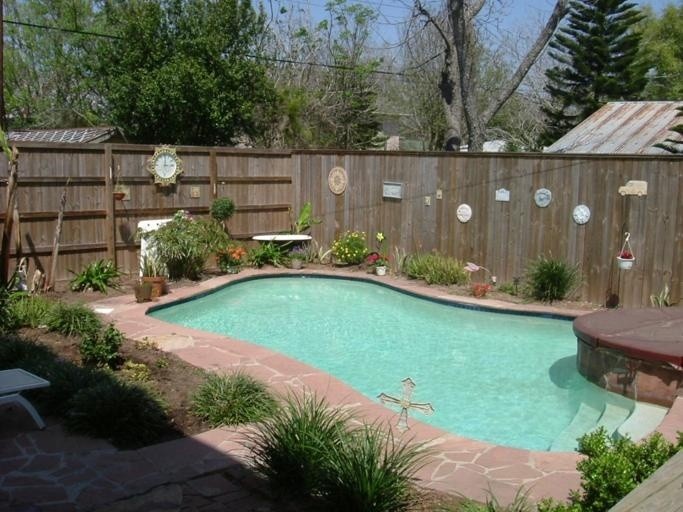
[251, 234, 313, 242]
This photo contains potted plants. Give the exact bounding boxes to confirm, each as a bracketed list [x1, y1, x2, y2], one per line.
[134, 250, 169, 303]
[287, 253, 306, 270]
[111, 170, 125, 201]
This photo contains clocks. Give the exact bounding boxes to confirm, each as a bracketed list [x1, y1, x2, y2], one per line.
[145, 145, 185, 189]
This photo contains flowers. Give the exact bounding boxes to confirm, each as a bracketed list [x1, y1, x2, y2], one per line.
[224, 241, 246, 264]
[618, 249, 633, 259]
[367, 253, 392, 266]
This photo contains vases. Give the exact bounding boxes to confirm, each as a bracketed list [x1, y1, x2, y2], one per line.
[225, 264, 239, 273]
[616, 256, 635, 270]
[375, 266, 386, 276]
